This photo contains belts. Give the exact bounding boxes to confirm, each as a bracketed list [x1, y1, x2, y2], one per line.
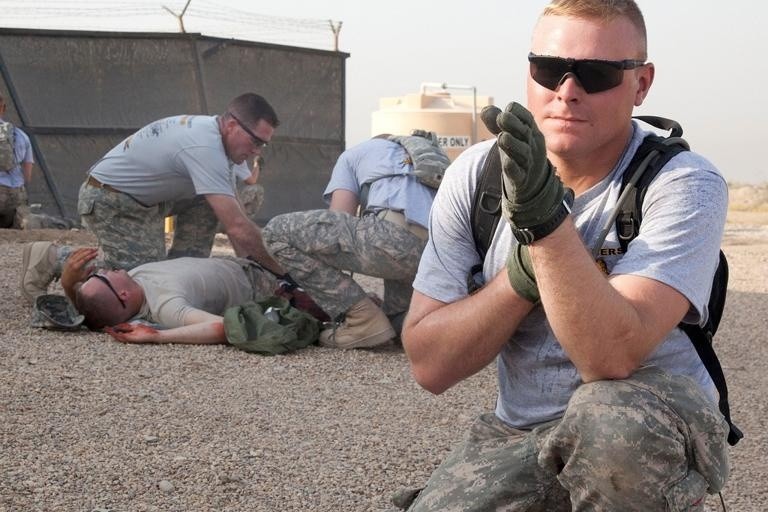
[83, 172, 126, 195]
[372, 203, 431, 246]
[0, 185, 26, 193]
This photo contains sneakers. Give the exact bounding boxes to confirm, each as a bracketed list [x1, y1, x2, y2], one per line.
[316, 293, 399, 352]
[12, 202, 46, 232]
[18, 237, 63, 309]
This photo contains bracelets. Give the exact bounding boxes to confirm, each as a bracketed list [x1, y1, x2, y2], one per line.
[511, 188, 575, 246]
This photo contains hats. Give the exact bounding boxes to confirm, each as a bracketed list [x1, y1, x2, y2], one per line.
[27, 291, 88, 334]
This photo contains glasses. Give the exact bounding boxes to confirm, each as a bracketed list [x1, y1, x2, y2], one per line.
[86, 272, 128, 310]
[230, 113, 271, 149]
[525, 48, 649, 95]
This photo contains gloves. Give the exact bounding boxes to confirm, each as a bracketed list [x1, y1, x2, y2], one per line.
[505, 238, 548, 307]
[475, 98, 567, 230]
[253, 153, 267, 167]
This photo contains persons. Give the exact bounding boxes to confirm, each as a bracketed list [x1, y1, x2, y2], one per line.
[389, 0, 744, 512]
[260, 129, 451, 349]
[216, 155, 265, 234]
[21, 92, 297, 308]
[61, 246, 383, 345]
[0, 93, 35, 230]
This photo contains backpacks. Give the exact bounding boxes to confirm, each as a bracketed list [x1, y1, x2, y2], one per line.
[0, 120, 19, 172]
[366, 128, 452, 192]
[468, 108, 733, 361]
